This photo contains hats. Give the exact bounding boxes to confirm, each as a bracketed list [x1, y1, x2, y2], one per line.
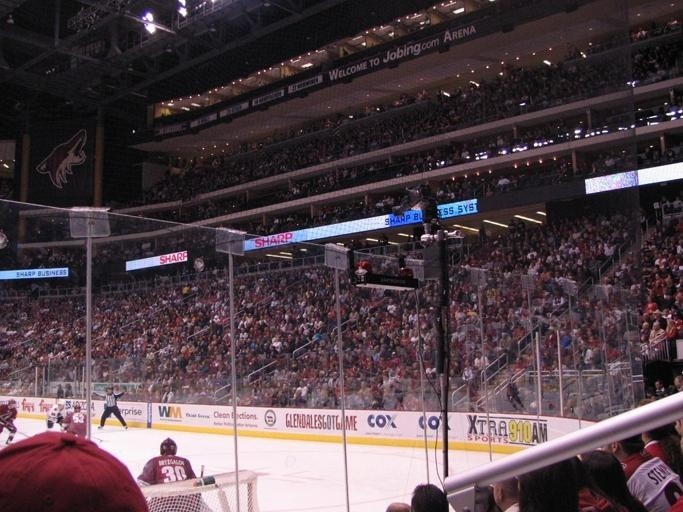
[0, 431, 148, 512]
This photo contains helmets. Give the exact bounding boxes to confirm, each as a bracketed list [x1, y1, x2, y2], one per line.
[8, 399, 15, 404]
[160, 438, 176, 455]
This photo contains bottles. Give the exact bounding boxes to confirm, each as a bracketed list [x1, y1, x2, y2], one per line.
[191, 475, 216, 488]
